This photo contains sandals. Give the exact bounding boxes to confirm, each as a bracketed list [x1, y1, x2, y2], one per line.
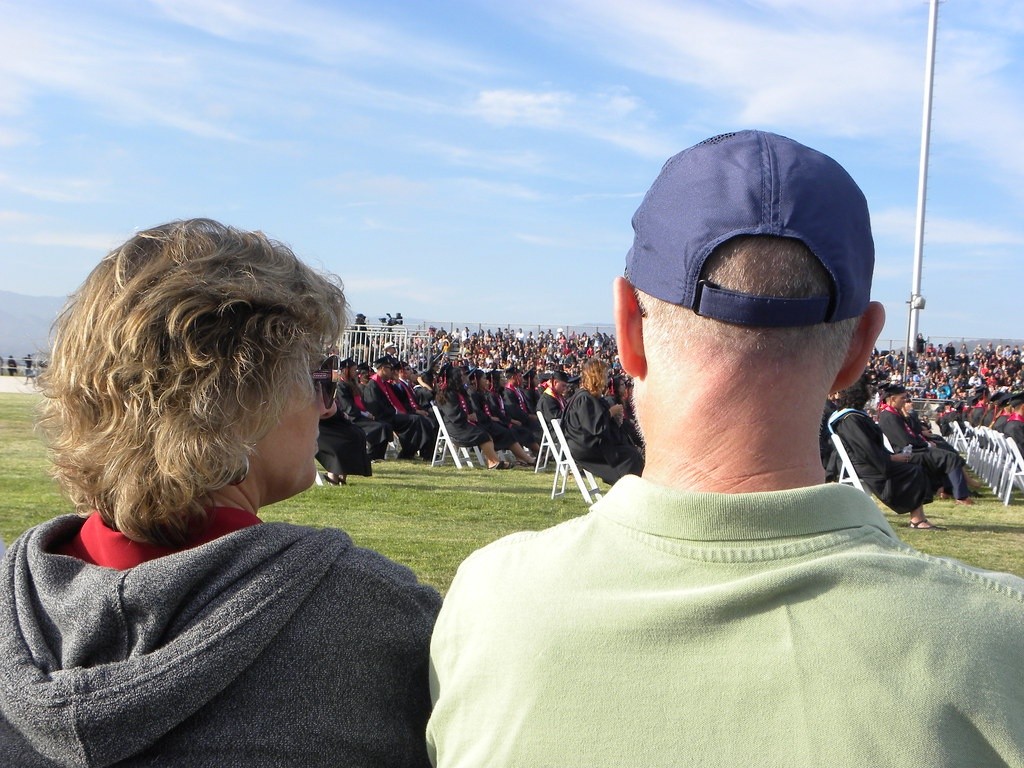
[910, 519, 947, 532]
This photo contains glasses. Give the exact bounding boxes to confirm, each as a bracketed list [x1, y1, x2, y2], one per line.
[403, 367, 410, 371]
[381, 365, 392, 370]
[308, 355, 341, 411]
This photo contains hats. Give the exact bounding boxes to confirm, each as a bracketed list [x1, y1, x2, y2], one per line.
[935, 384, 1024, 414]
[550, 370, 581, 390]
[382, 341, 399, 350]
[373, 356, 410, 371]
[437, 364, 549, 392]
[625, 128, 875, 327]
[340, 356, 375, 376]
[876, 382, 906, 399]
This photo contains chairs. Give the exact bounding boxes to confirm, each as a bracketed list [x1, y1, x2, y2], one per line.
[428, 403, 602, 508]
[928, 419, 1024, 504]
[830, 436, 865, 500]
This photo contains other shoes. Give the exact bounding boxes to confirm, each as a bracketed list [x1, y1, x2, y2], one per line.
[968, 489, 984, 497]
[535, 450, 556, 461]
[487, 460, 515, 470]
[323, 472, 349, 486]
[514, 456, 543, 467]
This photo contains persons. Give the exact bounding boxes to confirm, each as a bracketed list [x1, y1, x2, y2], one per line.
[819, 334, 1024, 531]
[0, 354, 33, 376]
[426, 129, 1024, 768]
[0, 219, 442, 768]
[314, 313, 645, 488]
